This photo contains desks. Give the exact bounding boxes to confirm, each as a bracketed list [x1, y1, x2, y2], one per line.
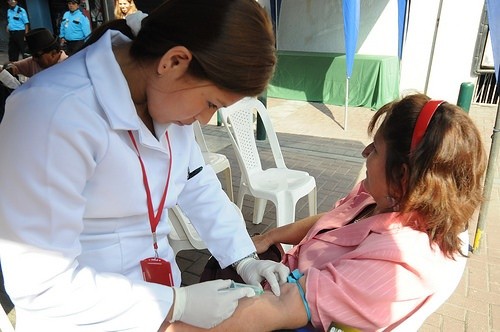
[267, 50, 400, 111]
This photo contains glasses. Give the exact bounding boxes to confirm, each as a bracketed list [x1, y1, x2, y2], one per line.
[30, 52, 45, 57]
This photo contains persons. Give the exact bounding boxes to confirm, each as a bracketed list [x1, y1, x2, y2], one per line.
[159, 93, 488, 332]
[6, 0, 28, 61]
[119, 0, 137, 19]
[0, 28, 69, 78]
[0, 0, 290, 332]
[59, 0, 91, 55]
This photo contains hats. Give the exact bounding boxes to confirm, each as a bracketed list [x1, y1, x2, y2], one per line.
[24, 28, 57, 54]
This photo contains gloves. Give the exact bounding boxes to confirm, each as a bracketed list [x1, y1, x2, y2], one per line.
[168, 279, 255, 329]
[236, 257, 290, 297]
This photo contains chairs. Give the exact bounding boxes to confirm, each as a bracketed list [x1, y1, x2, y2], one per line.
[193, 119, 233, 202]
[390, 232, 469, 332]
[219, 97, 317, 227]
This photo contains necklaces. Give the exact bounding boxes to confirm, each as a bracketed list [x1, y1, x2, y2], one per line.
[353, 205, 378, 225]
